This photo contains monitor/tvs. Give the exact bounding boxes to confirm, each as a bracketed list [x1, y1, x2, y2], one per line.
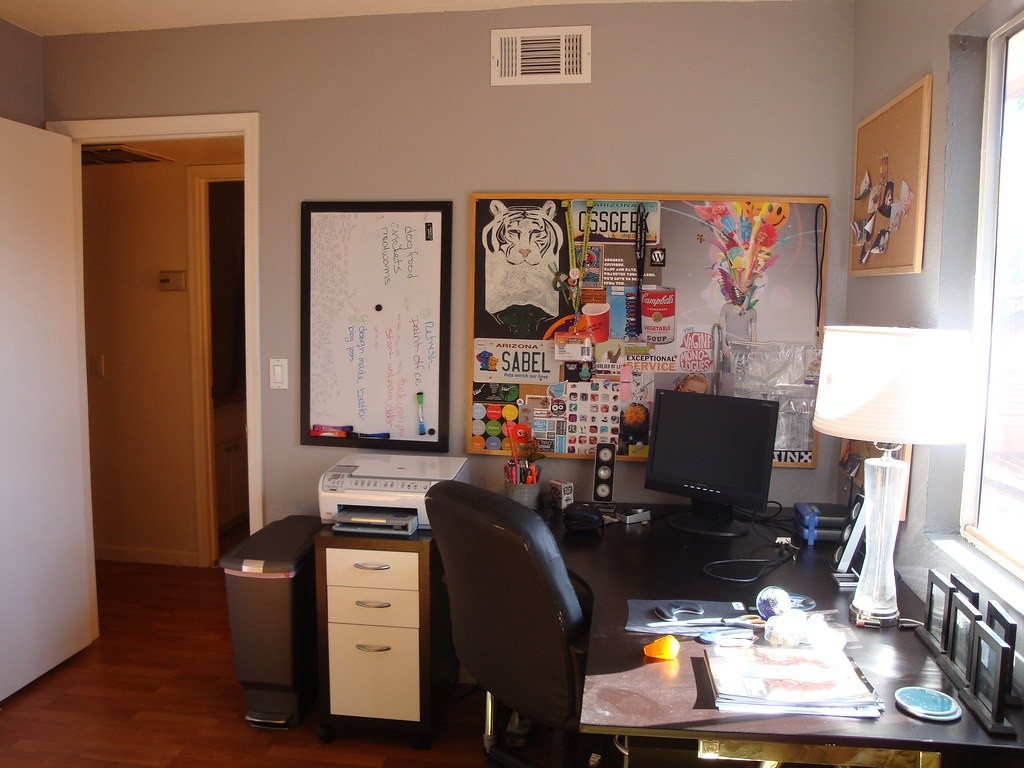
[643, 389, 779, 537]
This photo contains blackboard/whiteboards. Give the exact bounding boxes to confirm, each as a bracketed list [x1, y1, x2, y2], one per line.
[300, 198, 454, 455]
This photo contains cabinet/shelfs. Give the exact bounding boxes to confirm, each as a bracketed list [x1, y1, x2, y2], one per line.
[309, 532, 461, 755]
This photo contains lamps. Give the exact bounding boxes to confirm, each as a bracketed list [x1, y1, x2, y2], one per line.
[807, 323, 983, 633]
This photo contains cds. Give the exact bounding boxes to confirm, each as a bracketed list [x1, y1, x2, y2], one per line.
[788, 592, 816, 610]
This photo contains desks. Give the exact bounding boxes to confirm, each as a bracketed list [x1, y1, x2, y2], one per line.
[560, 500, 1024, 768]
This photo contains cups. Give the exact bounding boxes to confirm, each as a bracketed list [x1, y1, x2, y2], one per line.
[591, 405, 598, 413]
[582, 303, 610, 342]
[506, 481, 541, 511]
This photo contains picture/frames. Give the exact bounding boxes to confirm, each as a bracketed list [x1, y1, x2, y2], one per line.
[299, 201, 455, 455]
[846, 72, 934, 279]
[465, 189, 830, 469]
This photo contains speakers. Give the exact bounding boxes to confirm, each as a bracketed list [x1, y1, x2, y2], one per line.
[592, 443, 616, 512]
[830, 494, 866, 586]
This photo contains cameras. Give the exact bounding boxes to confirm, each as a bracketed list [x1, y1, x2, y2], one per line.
[616, 505, 651, 524]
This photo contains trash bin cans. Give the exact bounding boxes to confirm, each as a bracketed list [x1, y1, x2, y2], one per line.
[217, 514, 323, 731]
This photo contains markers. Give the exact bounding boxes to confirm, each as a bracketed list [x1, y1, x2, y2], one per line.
[416, 392, 427, 436]
[308, 423, 391, 439]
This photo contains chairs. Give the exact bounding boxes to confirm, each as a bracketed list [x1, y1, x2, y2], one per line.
[423, 478, 590, 768]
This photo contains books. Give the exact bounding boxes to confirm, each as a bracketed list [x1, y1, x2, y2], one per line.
[704, 643, 886, 718]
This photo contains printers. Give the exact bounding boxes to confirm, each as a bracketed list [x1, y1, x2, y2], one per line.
[318, 452, 471, 535]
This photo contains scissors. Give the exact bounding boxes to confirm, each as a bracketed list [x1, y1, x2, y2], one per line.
[686, 614, 767, 629]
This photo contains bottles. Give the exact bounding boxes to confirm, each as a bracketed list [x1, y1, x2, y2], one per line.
[580, 363, 590, 381]
[601, 405, 608, 412]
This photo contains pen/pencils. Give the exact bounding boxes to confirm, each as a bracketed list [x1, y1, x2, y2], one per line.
[497, 416, 543, 485]
